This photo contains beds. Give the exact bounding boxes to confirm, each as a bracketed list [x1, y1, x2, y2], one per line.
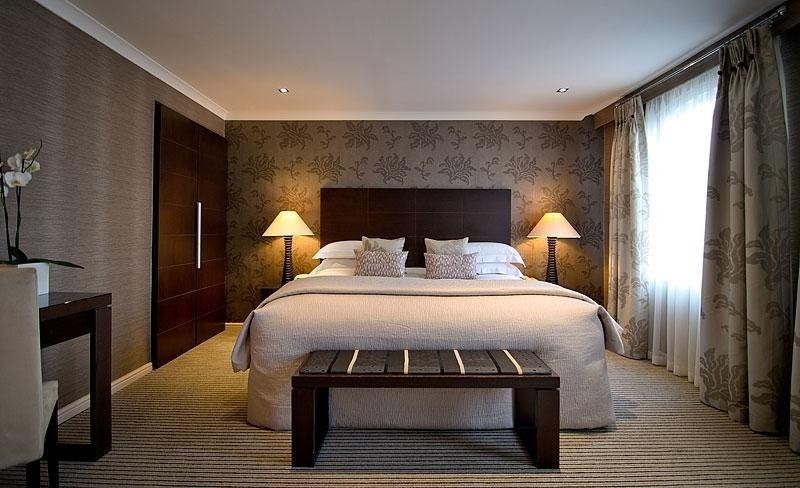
[244, 192, 623, 430]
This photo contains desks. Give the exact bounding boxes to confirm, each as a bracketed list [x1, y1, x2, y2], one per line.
[34, 290, 116, 463]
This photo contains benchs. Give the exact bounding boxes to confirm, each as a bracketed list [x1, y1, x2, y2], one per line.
[285, 349, 561, 470]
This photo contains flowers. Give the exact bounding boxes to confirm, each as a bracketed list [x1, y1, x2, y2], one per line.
[0, 144, 85, 269]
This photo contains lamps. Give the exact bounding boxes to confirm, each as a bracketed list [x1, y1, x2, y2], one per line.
[261, 209, 314, 289]
[527, 212, 582, 286]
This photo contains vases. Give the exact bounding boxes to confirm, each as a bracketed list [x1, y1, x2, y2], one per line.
[3, 263, 52, 295]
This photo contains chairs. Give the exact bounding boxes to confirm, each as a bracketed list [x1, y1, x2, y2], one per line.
[0, 263, 62, 488]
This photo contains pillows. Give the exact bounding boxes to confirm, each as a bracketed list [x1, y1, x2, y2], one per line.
[307, 237, 528, 282]
